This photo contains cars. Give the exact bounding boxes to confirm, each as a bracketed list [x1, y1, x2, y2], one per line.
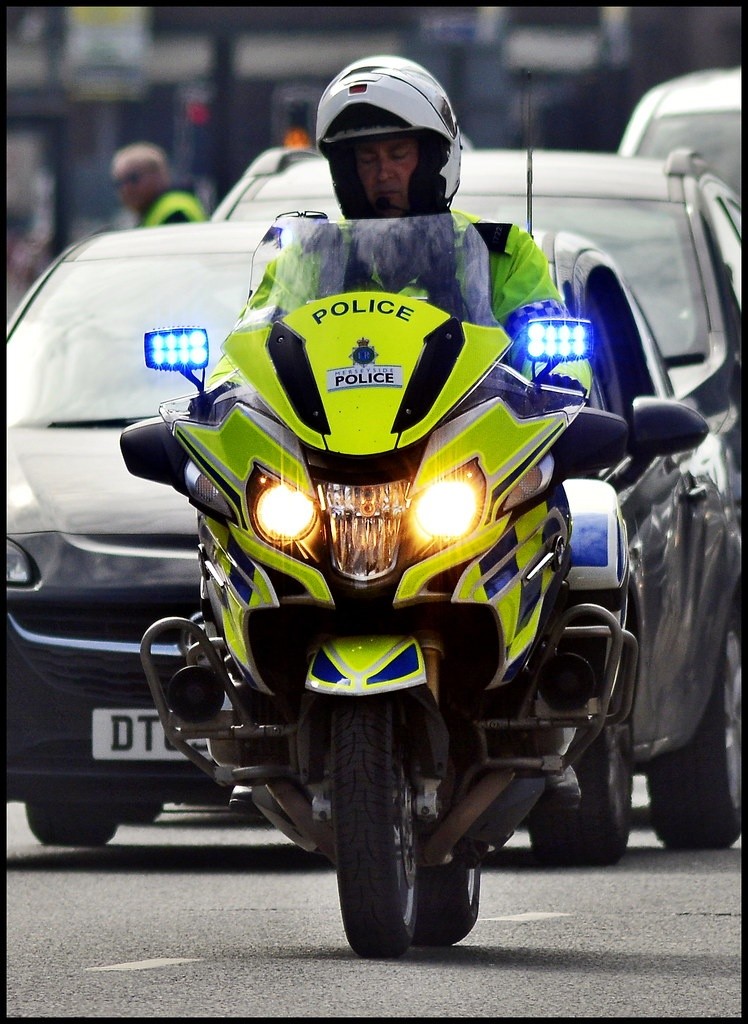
[6, 208, 743, 870]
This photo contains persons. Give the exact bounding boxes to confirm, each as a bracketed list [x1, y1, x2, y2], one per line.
[202, 53, 593, 814]
[108, 140, 206, 228]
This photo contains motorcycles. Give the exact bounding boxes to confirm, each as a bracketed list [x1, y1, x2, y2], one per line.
[118, 212, 712, 962]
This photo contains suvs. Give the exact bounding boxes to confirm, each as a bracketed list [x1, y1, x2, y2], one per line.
[211, 145, 741, 459]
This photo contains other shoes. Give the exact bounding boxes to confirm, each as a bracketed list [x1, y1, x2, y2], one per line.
[547, 767, 581, 813]
[229, 785, 261, 817]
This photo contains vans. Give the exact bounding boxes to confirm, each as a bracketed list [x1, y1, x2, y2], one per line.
[620, 64, 741, 232]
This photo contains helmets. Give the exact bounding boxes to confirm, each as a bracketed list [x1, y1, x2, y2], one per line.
[315, 56, 461, 218]
